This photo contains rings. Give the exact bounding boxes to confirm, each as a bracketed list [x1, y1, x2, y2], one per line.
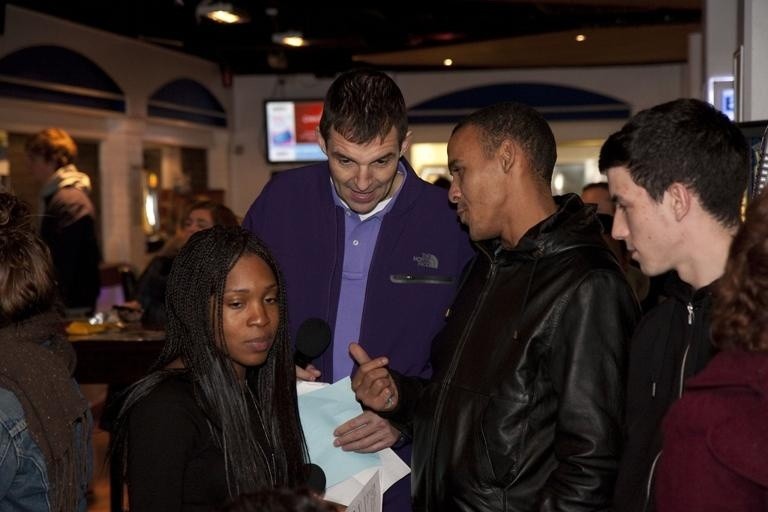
[385, 398, 392, 409]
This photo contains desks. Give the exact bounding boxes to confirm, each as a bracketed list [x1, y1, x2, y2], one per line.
[71, 329, 166, 512]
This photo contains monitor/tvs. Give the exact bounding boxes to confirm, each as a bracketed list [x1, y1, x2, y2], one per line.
[263, 97, 329, 164]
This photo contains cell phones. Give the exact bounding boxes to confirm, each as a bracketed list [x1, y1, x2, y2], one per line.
[113, 305, 141, 313]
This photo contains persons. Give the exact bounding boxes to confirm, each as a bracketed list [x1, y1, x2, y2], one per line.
[349, 98, 648, 512]
[106, 220, 348, 512]
[24, 127, 106, 308]
[647, 180, 768, 512]
[597, 213, 657, 316]
[0, 181, 96, 512]
[242, 68, 476, 512]
[597, 95, 755, 512]
[581, 181, 617, 215]
[117, 200, 238, 331]
